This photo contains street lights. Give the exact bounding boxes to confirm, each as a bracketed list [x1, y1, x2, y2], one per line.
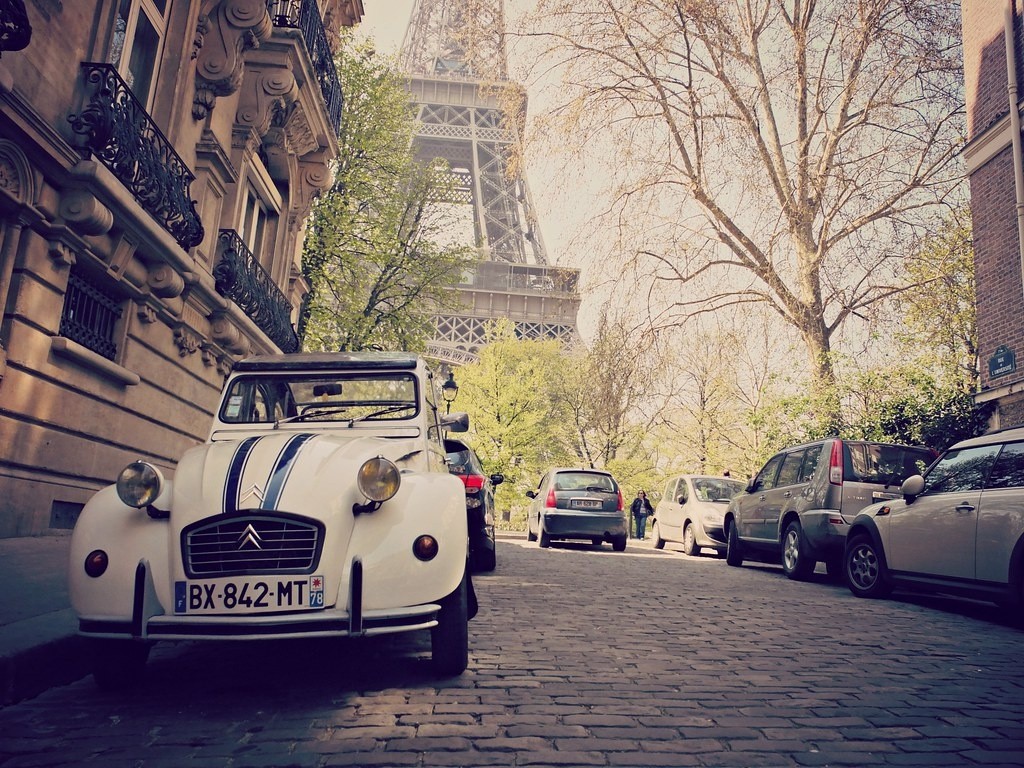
[442, 373, 459, 440]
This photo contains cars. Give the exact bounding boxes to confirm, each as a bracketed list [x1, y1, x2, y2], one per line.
[432, 439, 504, 571]
[525, 468, 628, 551]
[69, 351, 478, 696]
[651, 475, 748, 557]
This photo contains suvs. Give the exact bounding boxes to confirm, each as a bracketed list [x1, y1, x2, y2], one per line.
[841, 424, 1024, 620]
[723, 436, 955, 580]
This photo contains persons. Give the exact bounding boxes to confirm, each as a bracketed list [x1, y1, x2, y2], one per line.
[630, 490, 654, 540]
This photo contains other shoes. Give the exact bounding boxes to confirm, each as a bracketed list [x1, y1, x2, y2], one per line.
[641, 538, 644, 541]
